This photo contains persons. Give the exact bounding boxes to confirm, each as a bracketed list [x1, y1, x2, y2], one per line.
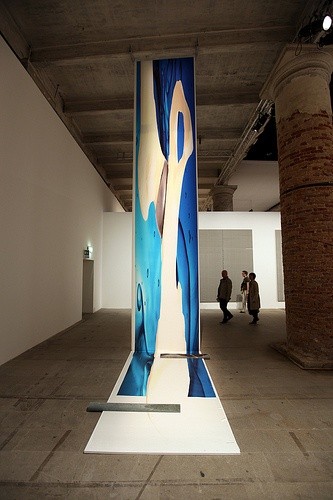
[216, 270, 233, 324]
[240, 271, 249, 313]
[247, 273, 261, 325]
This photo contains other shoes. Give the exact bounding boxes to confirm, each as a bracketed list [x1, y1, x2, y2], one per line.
[240, 311, 245, 313]
[227, 314, 233, 321]
[256, 318, 259, 321]
[220, 321, 227, 323]
[249, 322, 256, 324]
